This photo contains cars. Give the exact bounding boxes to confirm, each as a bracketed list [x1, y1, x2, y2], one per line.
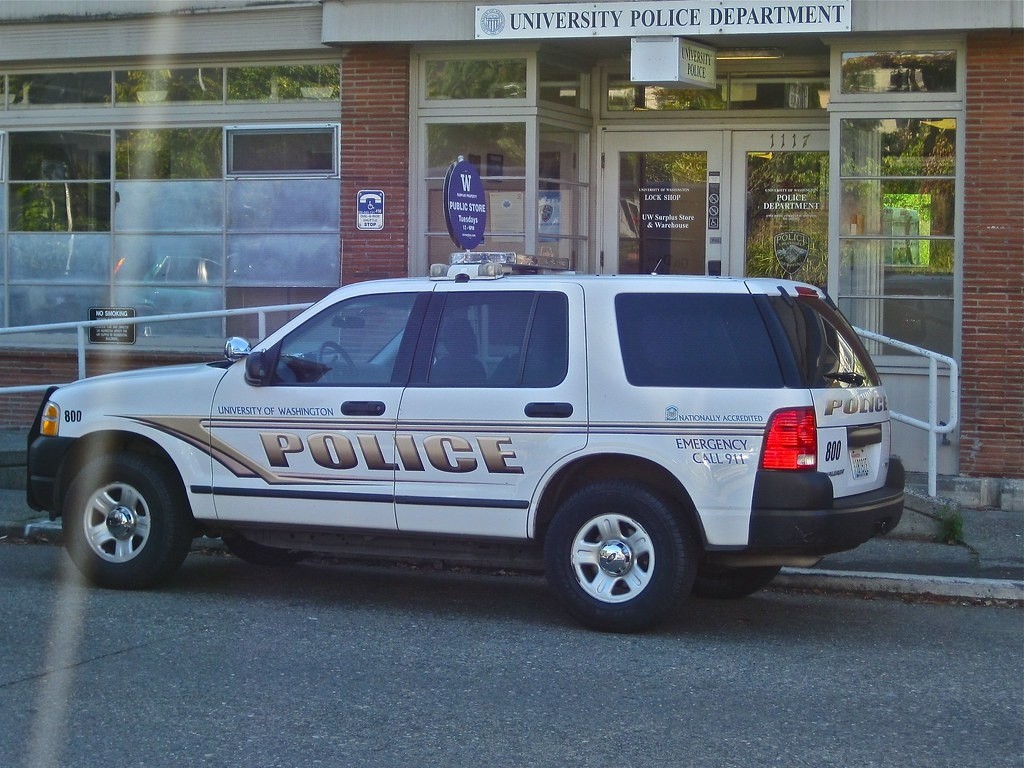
[103, 252, 225, 333]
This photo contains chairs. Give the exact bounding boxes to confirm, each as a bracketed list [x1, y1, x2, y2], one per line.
[429, 319, 488, 386]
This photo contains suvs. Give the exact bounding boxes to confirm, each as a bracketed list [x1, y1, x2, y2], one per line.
[28, 249, 910, 633]
